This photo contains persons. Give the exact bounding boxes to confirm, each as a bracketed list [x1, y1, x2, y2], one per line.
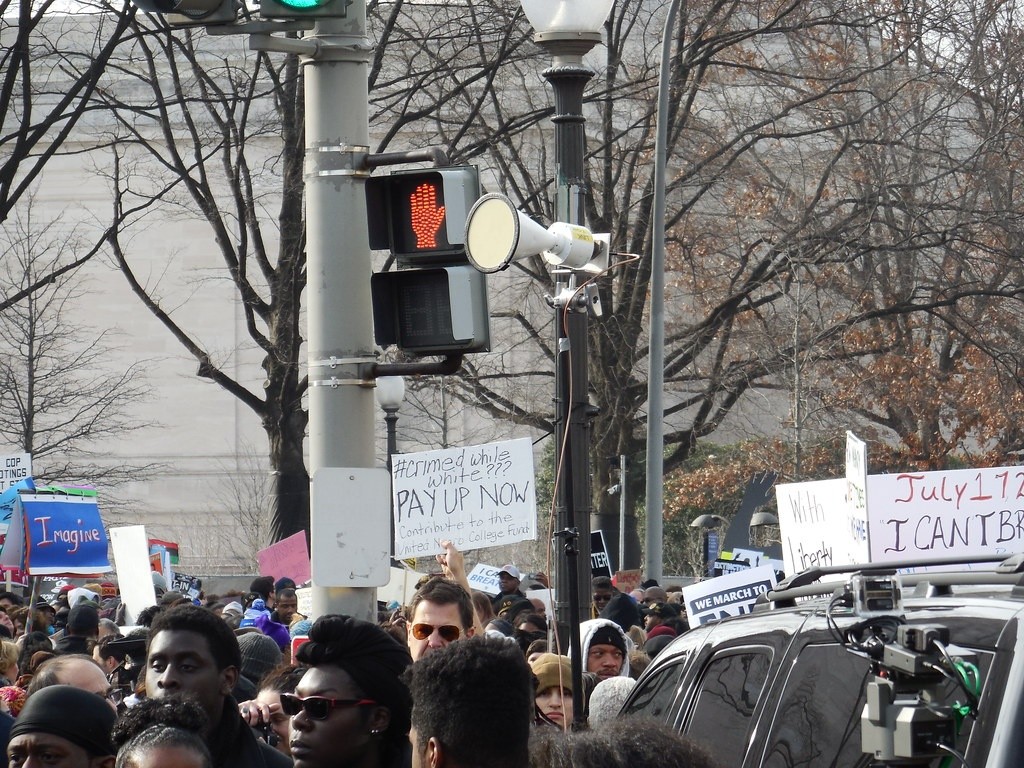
[0, 540, 726, 768]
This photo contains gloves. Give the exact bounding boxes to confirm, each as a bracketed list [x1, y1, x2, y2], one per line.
[115, 602, 125, 625]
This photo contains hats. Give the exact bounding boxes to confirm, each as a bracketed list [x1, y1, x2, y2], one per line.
[489, 594, 535, 637]
[641, 579, 683, 655]
[496, 564, 521, 581]
[589, 627, 626, 654]
[221, 576, 315, 681]
[23, 595, 56, 616]
[530, 653, 572, 698]
[529, 572, 548, 588]
[7, 684, 117, 757]
[151, 571, 166, 593]
[588, 676, 637, 728]
[50, 580, 116, 630]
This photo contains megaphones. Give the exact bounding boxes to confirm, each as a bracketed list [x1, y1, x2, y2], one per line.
[464, 193, 594, 273]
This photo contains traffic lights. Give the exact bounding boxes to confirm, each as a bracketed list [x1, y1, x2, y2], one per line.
[131, 0, 245, 27]
[261, 0, 347, 19]
[365, 162, 490, 358]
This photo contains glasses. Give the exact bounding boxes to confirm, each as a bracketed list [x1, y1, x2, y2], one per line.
[279, 694, 377, 721]
[592, 594, 612, 602]
[257, 711, 287, 727]
[410, 623, 464, 643]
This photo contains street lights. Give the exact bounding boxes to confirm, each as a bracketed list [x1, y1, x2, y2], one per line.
[519, 0, 619, 654]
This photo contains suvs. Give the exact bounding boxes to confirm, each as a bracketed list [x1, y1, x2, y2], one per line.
[613, 551, 1024, 768]
